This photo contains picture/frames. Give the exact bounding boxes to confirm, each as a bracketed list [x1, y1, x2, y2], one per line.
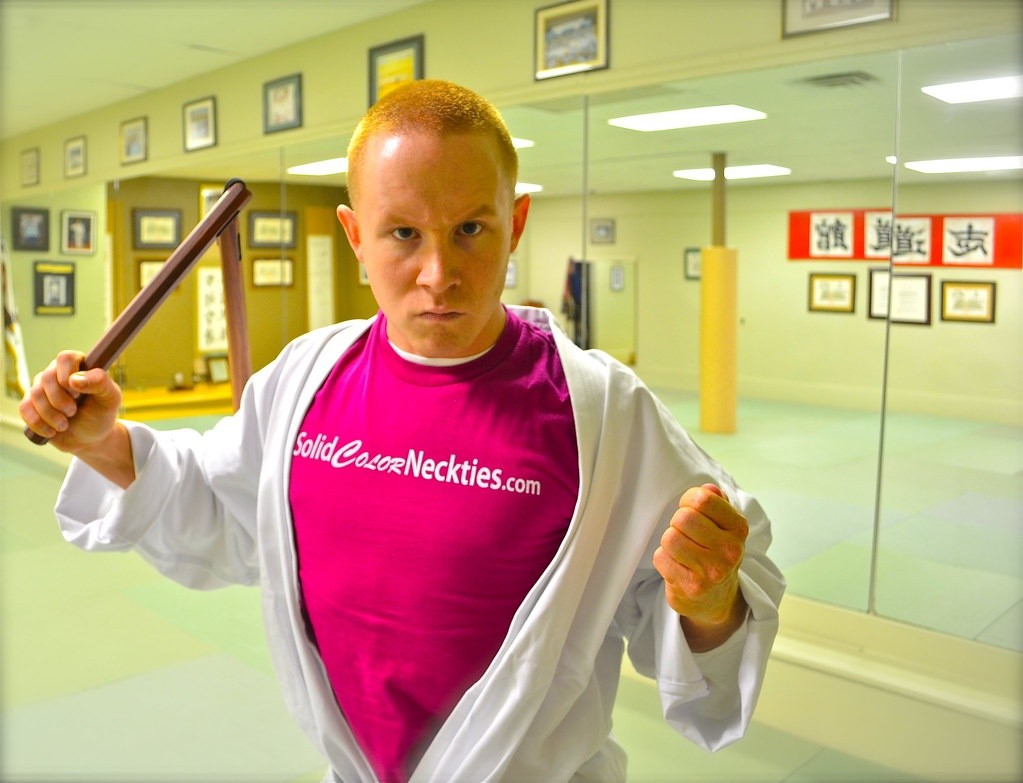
[206, 355, 232, 384]
[247, 206, 298, 251]
[133, 206, 181, 252]
[136, 259, 168, 290]
[61, 135, 86, 178]
[115, 115, 146, 165]
[252, 258, 294, 286]
[365, 33, 422, 111]
[535, 1, 609, 84]
[781, 0, 897, 41]
[181, 95, 217, 153]
[807, 274, 855, 314]
[10, 205, 50, 252]
[18, 147, 38, 187]
[684, 249, 702, 281]
[942, 281, 995, 324]
[35, 262, 76, 315]
[870, 269, 931, 327]
[260, 72, 302, 135]
[60, 210, 98, 257]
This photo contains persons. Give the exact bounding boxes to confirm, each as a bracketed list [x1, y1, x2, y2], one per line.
[20, 81, 785, 783]
